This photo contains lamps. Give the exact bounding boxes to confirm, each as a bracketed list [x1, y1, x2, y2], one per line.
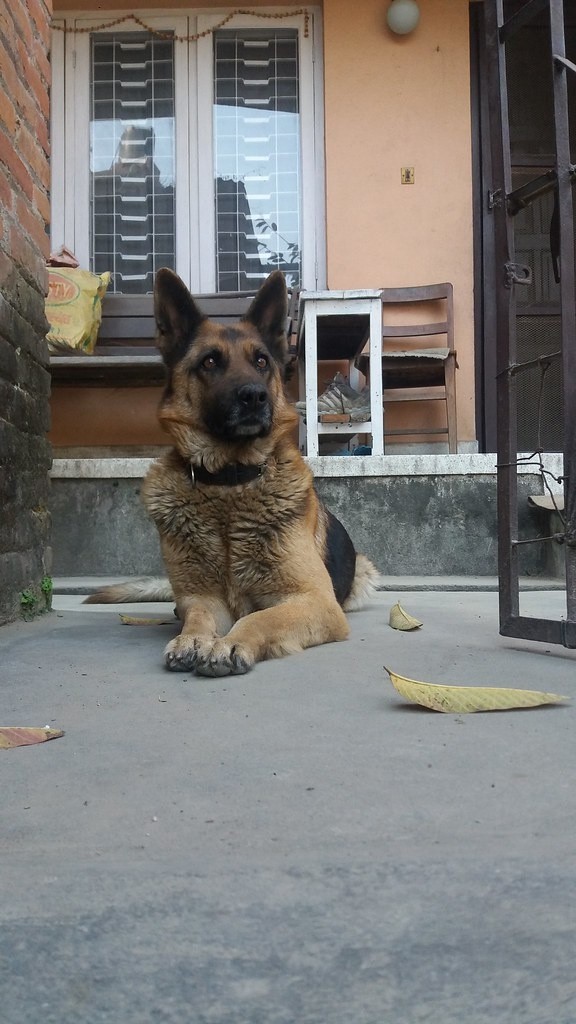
[386, 0, 421, 36]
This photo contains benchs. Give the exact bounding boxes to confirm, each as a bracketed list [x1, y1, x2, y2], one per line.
[50, 294, 296, 389]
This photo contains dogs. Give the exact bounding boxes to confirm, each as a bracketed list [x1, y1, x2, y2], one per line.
[80, 266, 386, 677]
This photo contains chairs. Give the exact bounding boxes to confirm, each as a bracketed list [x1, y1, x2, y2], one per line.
[353, 282, 457, 455]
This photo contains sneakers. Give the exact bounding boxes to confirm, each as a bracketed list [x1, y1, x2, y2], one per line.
[295, 371, 371, 423]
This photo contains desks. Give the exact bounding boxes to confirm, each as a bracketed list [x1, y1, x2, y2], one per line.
[296, 289, 384, 456]
